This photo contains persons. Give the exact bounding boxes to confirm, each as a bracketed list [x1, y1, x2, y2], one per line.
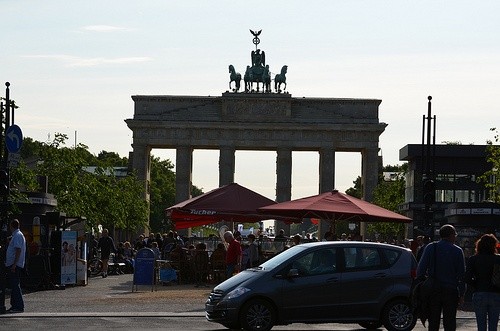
[257, 227, 426, 256]
[246, 234, 262, 266]
[94, 229, 118, 278]
[118, 242, 134, 274]
[61, 242, 76, 265]
[5, 219, 27, 313]
[224, 231, 242, 279]
[90, 235, 100, 255]
[467, 234, 500, 331]
[416, 224, 465, 331]
[255, 49, 261, 66]
[136, 230, 245, 286]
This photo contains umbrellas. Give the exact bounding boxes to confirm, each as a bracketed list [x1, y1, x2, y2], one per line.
[257, 190, 414, 235]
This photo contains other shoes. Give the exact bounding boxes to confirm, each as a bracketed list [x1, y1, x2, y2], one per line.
[105, 274, 107, 277]
[101, 273, 105, 277]
[6, 307, 24, 313]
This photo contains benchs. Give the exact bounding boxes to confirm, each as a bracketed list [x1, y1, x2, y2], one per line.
[107, 252, 128, 275]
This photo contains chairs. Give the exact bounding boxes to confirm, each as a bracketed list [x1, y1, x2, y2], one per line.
[154, 246, 227, 287]
[334, 248, 347, 272]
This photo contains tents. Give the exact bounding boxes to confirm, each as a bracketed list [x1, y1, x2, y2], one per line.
[166, 183, 277, 229]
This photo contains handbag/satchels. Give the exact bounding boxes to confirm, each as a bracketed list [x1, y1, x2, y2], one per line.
[420, 274, 438, 300]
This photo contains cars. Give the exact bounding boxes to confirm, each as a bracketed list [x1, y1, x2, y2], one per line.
[204, 241, 424, 331]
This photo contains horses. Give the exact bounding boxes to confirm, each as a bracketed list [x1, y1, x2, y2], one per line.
[228, 65, 242, 93]
[274, 65, 288, 92]
[244, 64, 271, 93]
